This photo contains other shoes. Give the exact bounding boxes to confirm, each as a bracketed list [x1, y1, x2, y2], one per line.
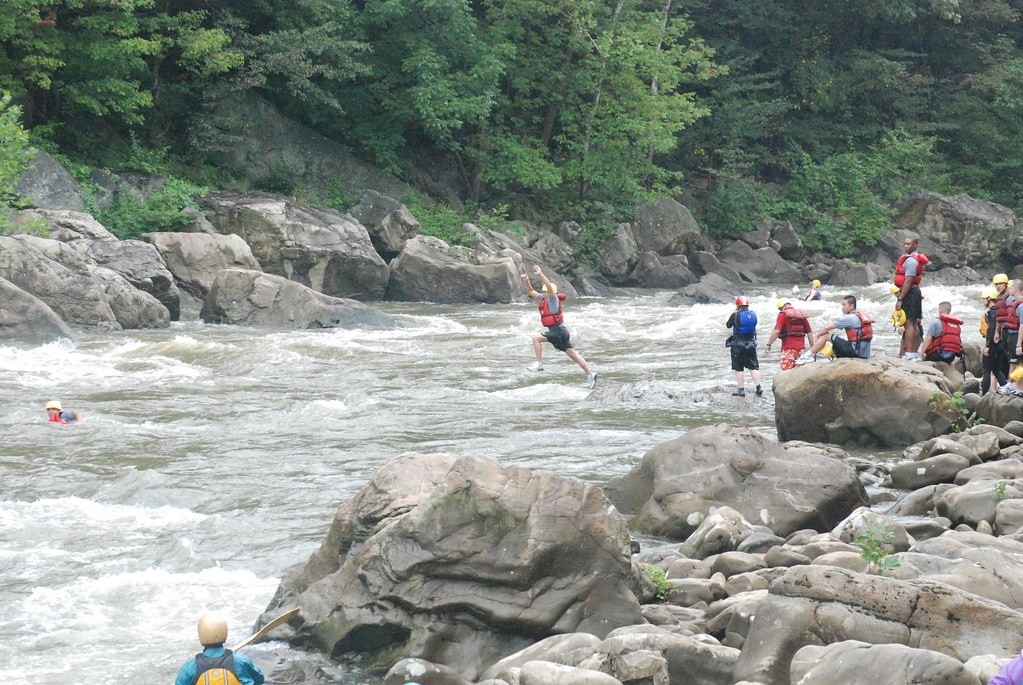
[997, 384, 1023, 397]
[901, 353, 920, 362]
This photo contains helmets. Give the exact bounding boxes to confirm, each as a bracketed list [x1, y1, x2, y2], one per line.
[199, 616, 228, 647]
[1009, 365, 1023, 381]
[892, 308, 906, 327]
[47, 400, 62, 412]
[890, 282, 901, 293]
[736, 297, 749, 306]
[821, 340, 834, 358]
[982, 291, 998, 300]
[776, 299, 791, 310]
[541, 282, 558, 294]
[994, 273, 1008, 284]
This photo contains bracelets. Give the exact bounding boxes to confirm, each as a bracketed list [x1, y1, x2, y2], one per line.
[1016, 344, 1022, 347]
[767, 344, 771, 346]
[897, 296, 903, 301]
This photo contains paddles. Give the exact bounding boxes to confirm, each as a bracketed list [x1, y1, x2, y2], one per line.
[792, 285, 801, 300]
[231, 607, 301, 652]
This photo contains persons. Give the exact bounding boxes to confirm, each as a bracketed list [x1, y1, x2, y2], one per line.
[797, 279, 821, 302]
[765, 297, 816, 371]
[996, 278, 1023, 397]
[899, 320, 923, 358]
[895, 236, 924, 358]
[521, 264, 597, 389]
[979, 289, 1008, 397]
[726, 295, 763, 396]
[46, 400, 69, 424]
[993, 272, 1010, 381]
[794, 295, 870, 364]
[175, 613, 265, 685]
[988, 648, 1023, 684]
[917, 302, 964, 364]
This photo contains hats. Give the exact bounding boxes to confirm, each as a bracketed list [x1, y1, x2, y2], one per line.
[812, 280, 821, 286]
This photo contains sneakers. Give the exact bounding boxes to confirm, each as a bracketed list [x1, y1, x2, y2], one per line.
[587, 372, 598, 389]
[794, 354, 816, 364]
[527, 362, 543, 371]
[756, 389, 763, 396]
[733, 389, 746, 396]
